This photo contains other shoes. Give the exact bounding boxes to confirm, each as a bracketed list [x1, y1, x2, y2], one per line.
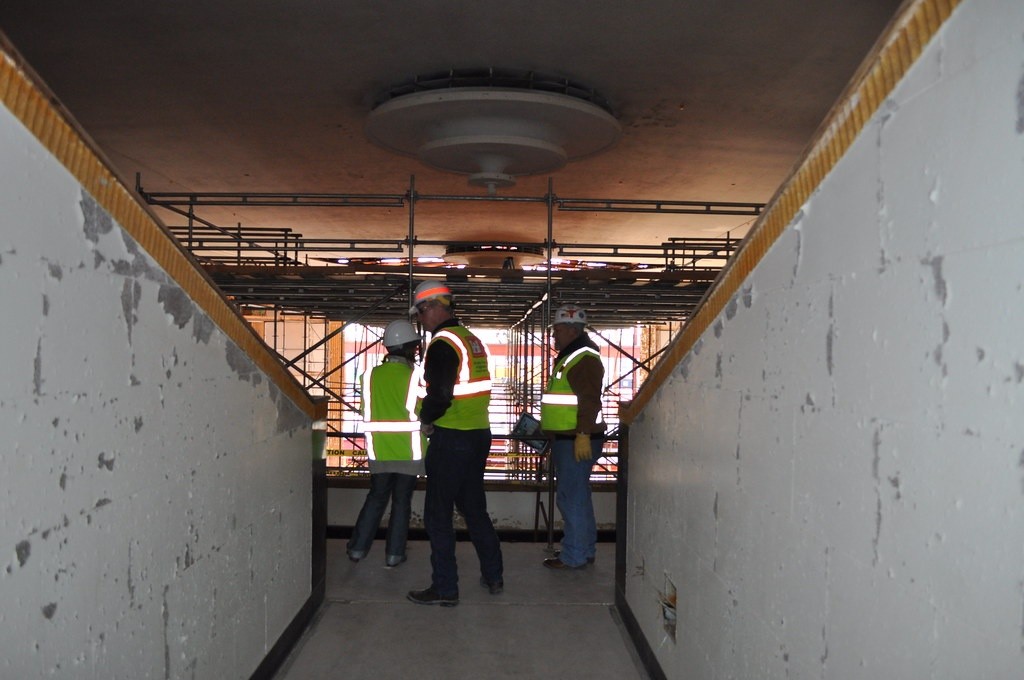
[387, 555, 406, 567]
[346, 550, 365, 562]
[479, 577, 503, 593]
[407, 584, 460, 607]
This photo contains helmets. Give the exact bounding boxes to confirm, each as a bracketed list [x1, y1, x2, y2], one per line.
[548, 304, 588, 327]
[409, 280, 451, 314]
[382, 319, 425, 346]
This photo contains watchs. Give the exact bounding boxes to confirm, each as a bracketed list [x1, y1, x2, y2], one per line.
[577, 431, 591, 436]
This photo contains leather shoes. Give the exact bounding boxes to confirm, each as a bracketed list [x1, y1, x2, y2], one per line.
[543, 557, 570, 569]
[552, 551, 594, 563]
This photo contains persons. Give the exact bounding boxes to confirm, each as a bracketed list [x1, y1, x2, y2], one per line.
[543, 304, 607, 571]
[345, 321, 428, 567]
[405, 278, 504, 607]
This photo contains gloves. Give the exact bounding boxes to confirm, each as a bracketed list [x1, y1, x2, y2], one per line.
[574, 433, 592, 461]
[420, 422, 435, 436]
